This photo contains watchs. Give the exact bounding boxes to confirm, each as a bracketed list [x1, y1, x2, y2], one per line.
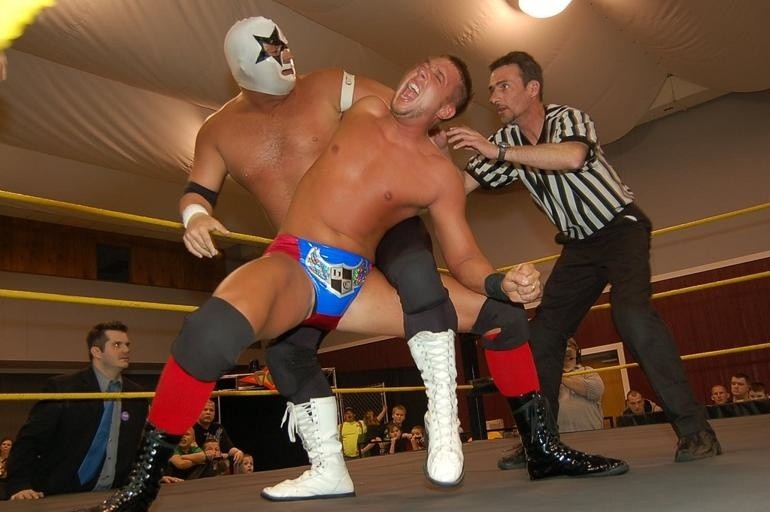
[498, 142, 510, 162]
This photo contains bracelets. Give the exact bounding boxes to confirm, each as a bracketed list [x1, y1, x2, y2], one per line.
[182, 204, 208, 229]
[485, 273, 508, 303]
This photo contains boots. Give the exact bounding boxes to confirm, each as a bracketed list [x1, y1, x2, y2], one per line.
[261, 396, 355, 501]
[91, 415, 183, 512]
[513, 391, 629, 480]
[407, 328, 465, 488]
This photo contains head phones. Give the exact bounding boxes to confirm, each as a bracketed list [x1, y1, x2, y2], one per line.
[576, 343, 581, 356]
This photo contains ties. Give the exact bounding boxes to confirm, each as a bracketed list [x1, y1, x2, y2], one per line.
[77, 384, 120, 486]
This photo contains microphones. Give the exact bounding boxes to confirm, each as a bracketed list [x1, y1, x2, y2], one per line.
[570, 356, 577, 360]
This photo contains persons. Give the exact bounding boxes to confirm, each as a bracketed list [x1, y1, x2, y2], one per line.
[92, 54, 629, 512]
[446, 52, 721, 470]
[179, 16, 465, 502]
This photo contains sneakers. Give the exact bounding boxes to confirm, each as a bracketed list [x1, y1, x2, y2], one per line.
[675, 430, 721, 461]
[498, 444, 524, 470]
